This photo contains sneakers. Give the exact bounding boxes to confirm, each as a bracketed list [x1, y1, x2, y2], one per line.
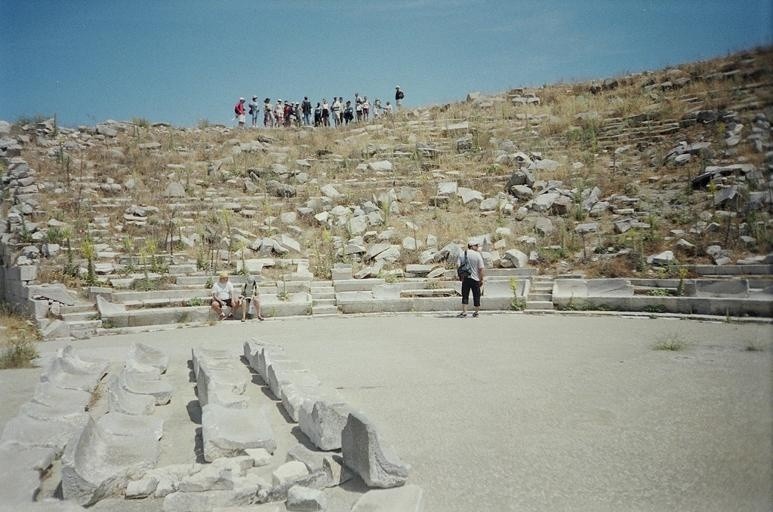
[472, 312, 478, 318]
[457, 313, 467, 318]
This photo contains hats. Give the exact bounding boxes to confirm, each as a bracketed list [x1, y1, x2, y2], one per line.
[467, 240, 480, 247]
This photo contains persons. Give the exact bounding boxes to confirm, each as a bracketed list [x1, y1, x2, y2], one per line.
[262, 93, 393, 128]
[455, 240, 485, 318]
[395, 84, 404, 111]
[235, 274, 266, 322]
[247, 95, 258, 128]
[232, 96, 246, 126]
[211, 271, 236, 320]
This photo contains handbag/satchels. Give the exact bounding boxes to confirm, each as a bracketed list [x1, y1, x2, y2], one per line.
[457, 250, 471, 281]
